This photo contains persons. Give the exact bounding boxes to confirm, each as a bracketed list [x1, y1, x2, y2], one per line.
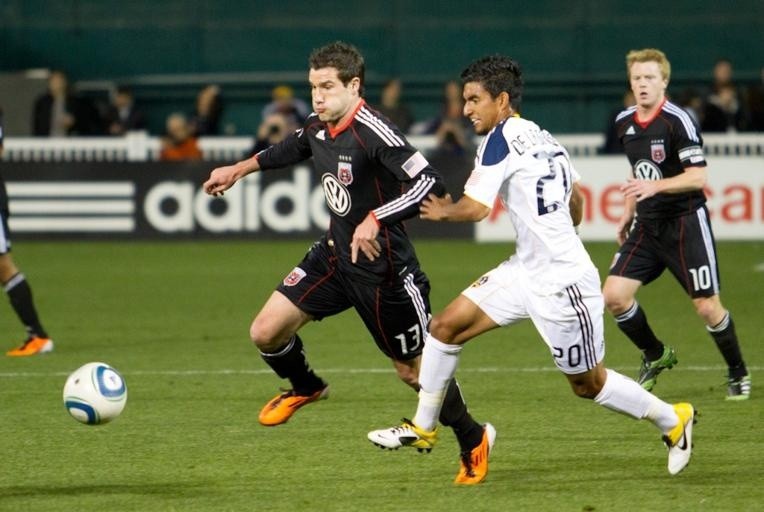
[600, 59, 764, 150]
[366, 55, 698, 475]
[603, 49, 751, 402]
[201, 40, 497, 485]
[1, 121, 54, 359]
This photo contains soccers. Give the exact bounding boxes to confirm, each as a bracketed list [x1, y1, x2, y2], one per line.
[63, 361, 127, 426]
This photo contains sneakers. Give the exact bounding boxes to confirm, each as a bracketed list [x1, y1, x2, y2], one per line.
[367, 417, 437, 454]
[456, 421, 496, 485]
[638, 344, 678, 391]
[725, 368, 752, 403]
[663, 403, 696, 478]
[258, 382, 329, 426]
[5, 337, 54, 356]
